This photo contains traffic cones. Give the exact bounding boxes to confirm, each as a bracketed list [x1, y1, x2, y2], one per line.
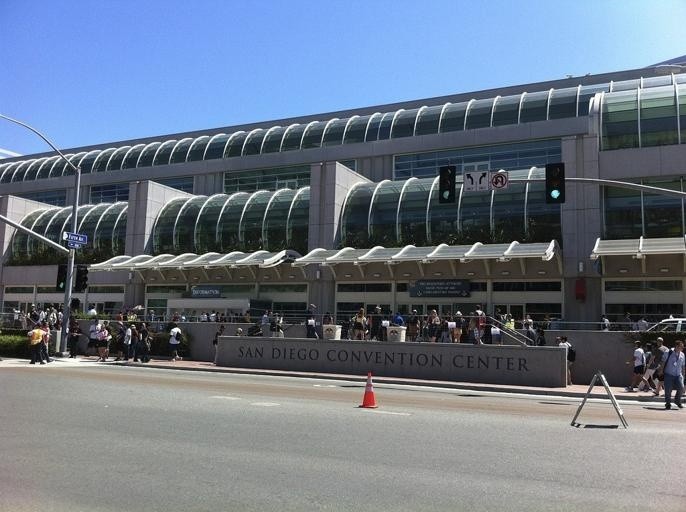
[358, 372, 380, 409]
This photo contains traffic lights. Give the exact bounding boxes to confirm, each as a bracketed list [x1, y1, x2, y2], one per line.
[439, 166, 456, 204]
[545, 162, 566, 204]
[55, 263, 67, 292]
[74, 264, 88, 293]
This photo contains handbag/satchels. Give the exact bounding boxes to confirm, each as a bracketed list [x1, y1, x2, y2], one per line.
[658, 368, 664, 381]
[567, 346, 576, 362]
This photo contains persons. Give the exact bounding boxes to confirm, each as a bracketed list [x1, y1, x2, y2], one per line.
[301, 303, 552, 346]
[626, 336, 684, 411]
[234, 324, 284, 338]
[600, 311, 684, 331]
[555, 335, 573, 385]
[213, 326, 226, 364]
[182, 307, 286, 324]
[13, 302, 182, 364]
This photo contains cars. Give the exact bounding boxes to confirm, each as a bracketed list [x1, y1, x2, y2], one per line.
[646, 318, 686, 331]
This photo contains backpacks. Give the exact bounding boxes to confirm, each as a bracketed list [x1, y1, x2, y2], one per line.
[175, 331, 183, 341]
